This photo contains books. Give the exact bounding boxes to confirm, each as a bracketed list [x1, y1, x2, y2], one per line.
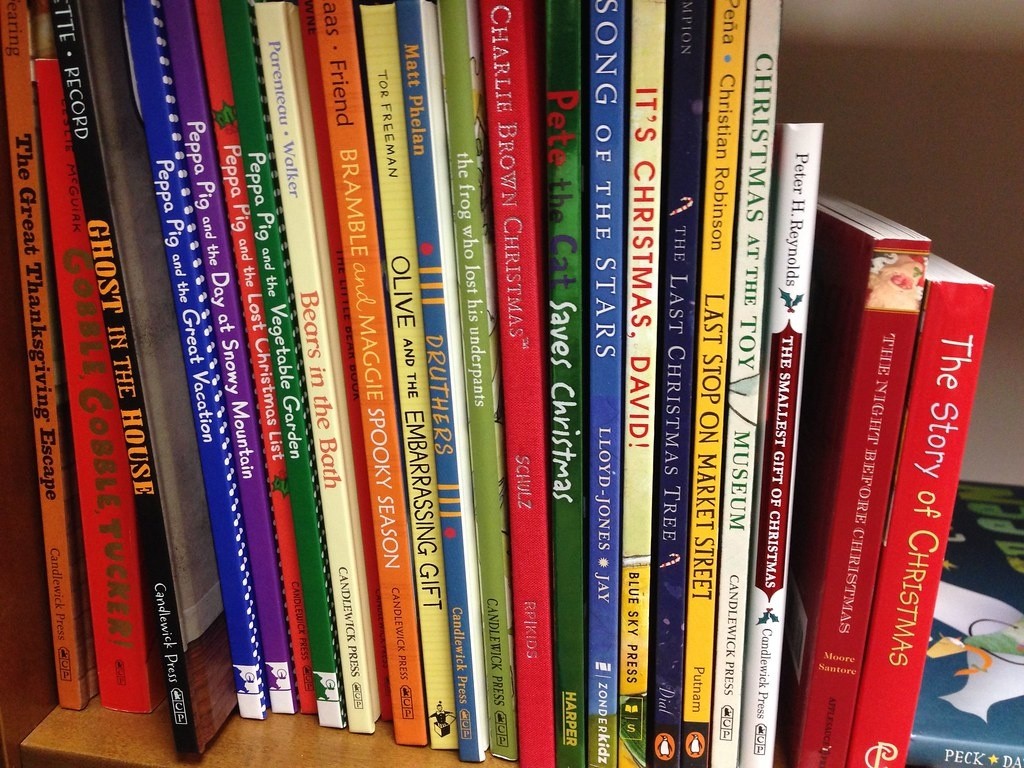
[2, 1, 1023, 768]
